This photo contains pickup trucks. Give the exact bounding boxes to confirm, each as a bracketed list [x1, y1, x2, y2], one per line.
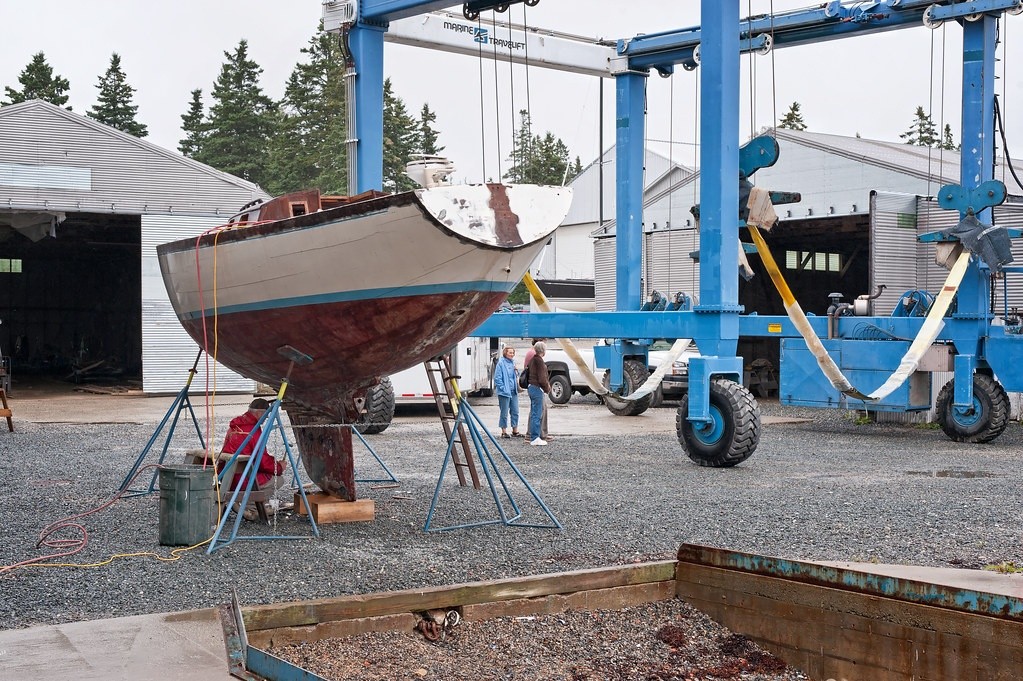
[535, 337, 701, 405]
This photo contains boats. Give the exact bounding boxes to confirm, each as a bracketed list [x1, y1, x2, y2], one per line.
[154, 153, 575, 419]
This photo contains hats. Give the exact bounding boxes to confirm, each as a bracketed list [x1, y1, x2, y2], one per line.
[249, 398, 272, 411]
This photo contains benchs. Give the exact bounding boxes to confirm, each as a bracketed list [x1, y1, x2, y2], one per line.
[183, 450, 269, 526]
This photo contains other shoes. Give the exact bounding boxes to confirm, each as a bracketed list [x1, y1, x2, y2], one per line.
[512, 432, 523, 437]
[541, 436, 554, 442]
[502, 433, 510, 438]
[530, 437, 547, 446]
[231, 501, 256, 521]
[525, 436, 530, 442]
[264, 504, 279, 516]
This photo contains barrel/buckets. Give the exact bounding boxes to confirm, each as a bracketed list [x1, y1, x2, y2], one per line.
[157, 463, 215, 547]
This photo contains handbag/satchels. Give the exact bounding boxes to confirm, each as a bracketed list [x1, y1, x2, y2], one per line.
[519, 365, 529, 389]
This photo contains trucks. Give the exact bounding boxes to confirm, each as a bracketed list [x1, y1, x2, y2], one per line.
[387, 336, 505, 408]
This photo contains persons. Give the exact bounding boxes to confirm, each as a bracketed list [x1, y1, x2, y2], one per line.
[527, 342, 551, 445]
[494, 347, 524, 438]
[217, 398, 287, 520]
[523, 337, 552, 440]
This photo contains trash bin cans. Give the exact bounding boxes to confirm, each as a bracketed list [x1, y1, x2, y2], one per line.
[157, 462, 219, 547]
